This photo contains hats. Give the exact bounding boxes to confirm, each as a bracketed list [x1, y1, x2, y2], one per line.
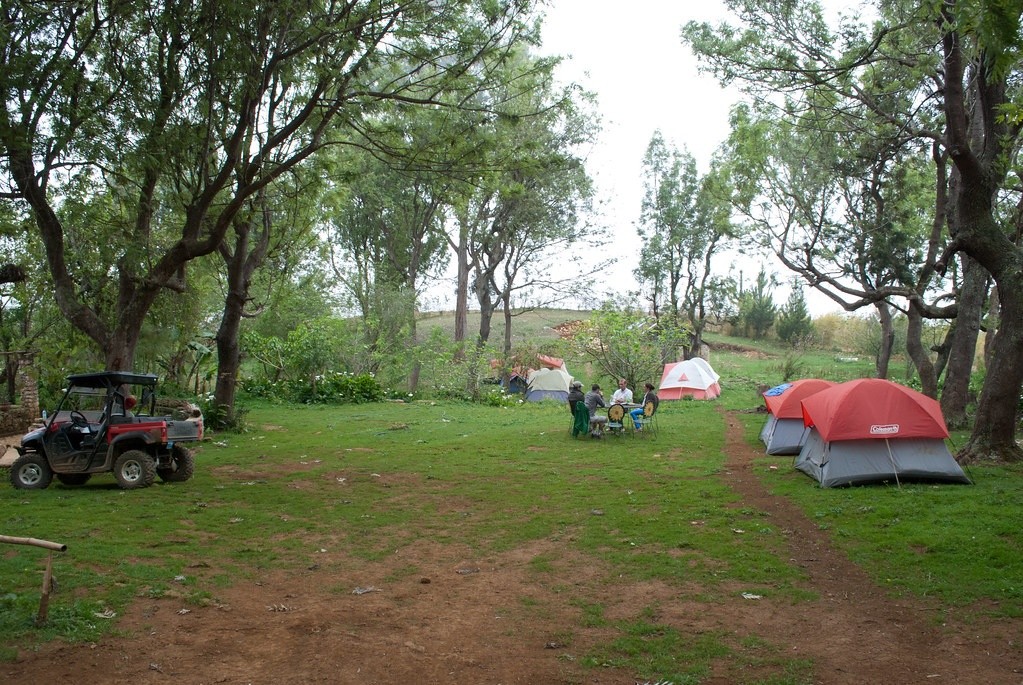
[592, 384, 601, 389]
[573, 381, 584, 387]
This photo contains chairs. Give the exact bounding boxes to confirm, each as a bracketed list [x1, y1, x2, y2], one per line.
[630, 400, 658, 441]
[642, 395, 659, 433]
[574, 400, 599, 441]
[602, 403, 626, 443]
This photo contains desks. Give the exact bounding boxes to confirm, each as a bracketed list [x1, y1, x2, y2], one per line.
[595, 402, 642, 434]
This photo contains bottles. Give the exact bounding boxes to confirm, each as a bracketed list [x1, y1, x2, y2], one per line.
[42, 409, 46, 419]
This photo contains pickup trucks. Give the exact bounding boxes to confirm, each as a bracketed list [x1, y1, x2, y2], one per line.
[9, 371, 204, 492]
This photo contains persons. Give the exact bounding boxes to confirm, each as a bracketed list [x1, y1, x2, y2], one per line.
[605, 379, 633, 432]
[584, 384, 608, 438]
[629, 383, 657, 433]
[568, 381, 585, 416]
[111, 396, 137, 418]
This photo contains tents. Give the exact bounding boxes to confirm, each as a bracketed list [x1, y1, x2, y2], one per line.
[758, 379, 836, 457]
[492, 352, 569, 380]
[526, 368, 574, 404]
[655, 360, 716, 400]
[499, 366, 535, 394]
[691, 357, 722, 397]
[792, 379, 976, 490]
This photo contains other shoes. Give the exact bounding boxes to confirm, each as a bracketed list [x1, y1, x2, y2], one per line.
[607, 430, 613, 433]
[592, 430, 603, 439]
[633, 424, 643, 432]
[619, 428, 625, 432]
